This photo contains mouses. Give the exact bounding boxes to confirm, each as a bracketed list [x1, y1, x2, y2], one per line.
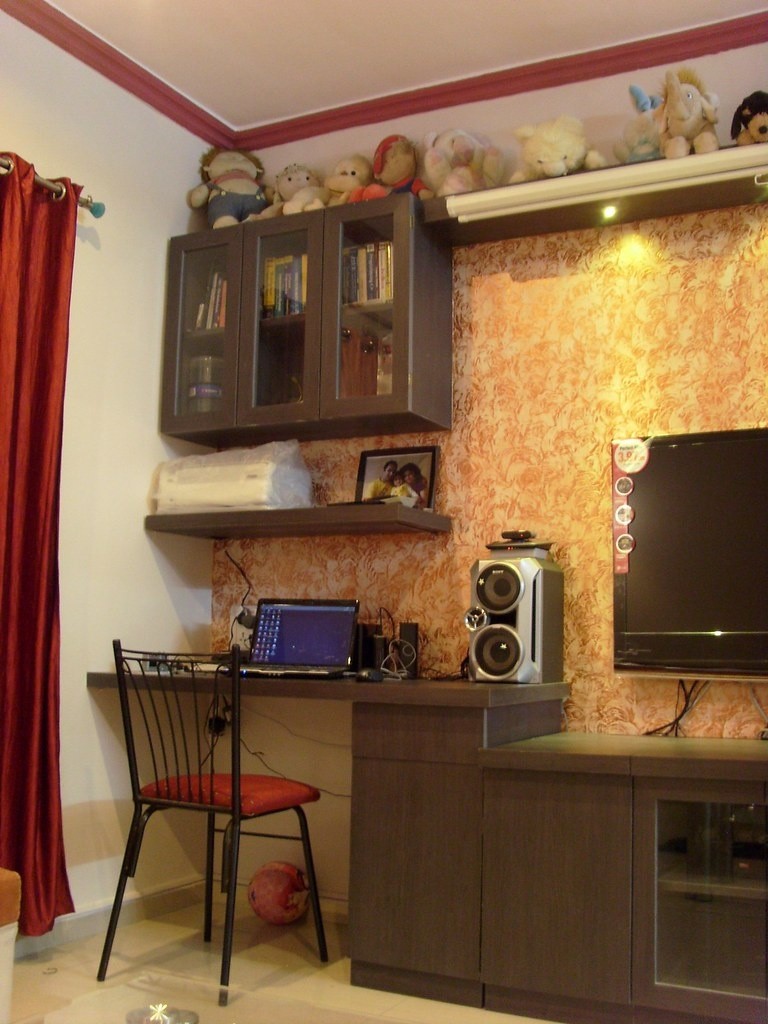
[356, 668, 383, 683]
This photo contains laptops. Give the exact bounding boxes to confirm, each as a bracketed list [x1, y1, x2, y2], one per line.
[220, 599, 360, 679]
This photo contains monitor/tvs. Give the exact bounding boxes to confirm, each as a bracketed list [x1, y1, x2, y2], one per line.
[612, 427, 768, 683]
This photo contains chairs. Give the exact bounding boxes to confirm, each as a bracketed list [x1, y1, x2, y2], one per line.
[96, 639, 329, 1006]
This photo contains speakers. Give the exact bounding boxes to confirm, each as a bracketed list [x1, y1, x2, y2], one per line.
[398, 623, 418, 678]
[464, 556, 564, 684]
[349, 623, 381, 672]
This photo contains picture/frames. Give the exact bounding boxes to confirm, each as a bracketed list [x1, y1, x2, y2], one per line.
[354, 445, 440, 512]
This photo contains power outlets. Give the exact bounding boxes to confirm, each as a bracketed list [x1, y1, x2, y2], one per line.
[228, 605, 259, 653]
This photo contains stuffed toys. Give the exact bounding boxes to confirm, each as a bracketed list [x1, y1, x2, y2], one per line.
[190, 67, 768, 227]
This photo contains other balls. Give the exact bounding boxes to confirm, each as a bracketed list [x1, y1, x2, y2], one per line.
[248, 862, 312, 924]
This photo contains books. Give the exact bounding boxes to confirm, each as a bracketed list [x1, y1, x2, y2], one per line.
[195, 239, 395, 334]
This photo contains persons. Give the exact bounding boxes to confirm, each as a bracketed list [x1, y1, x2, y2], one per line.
[361, 460, 428, 509]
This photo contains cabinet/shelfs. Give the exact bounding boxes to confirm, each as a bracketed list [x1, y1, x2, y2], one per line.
[161, 191, 452, 449]
[478, 731, 768, 1024]
[87, 671, 572, 1010]
